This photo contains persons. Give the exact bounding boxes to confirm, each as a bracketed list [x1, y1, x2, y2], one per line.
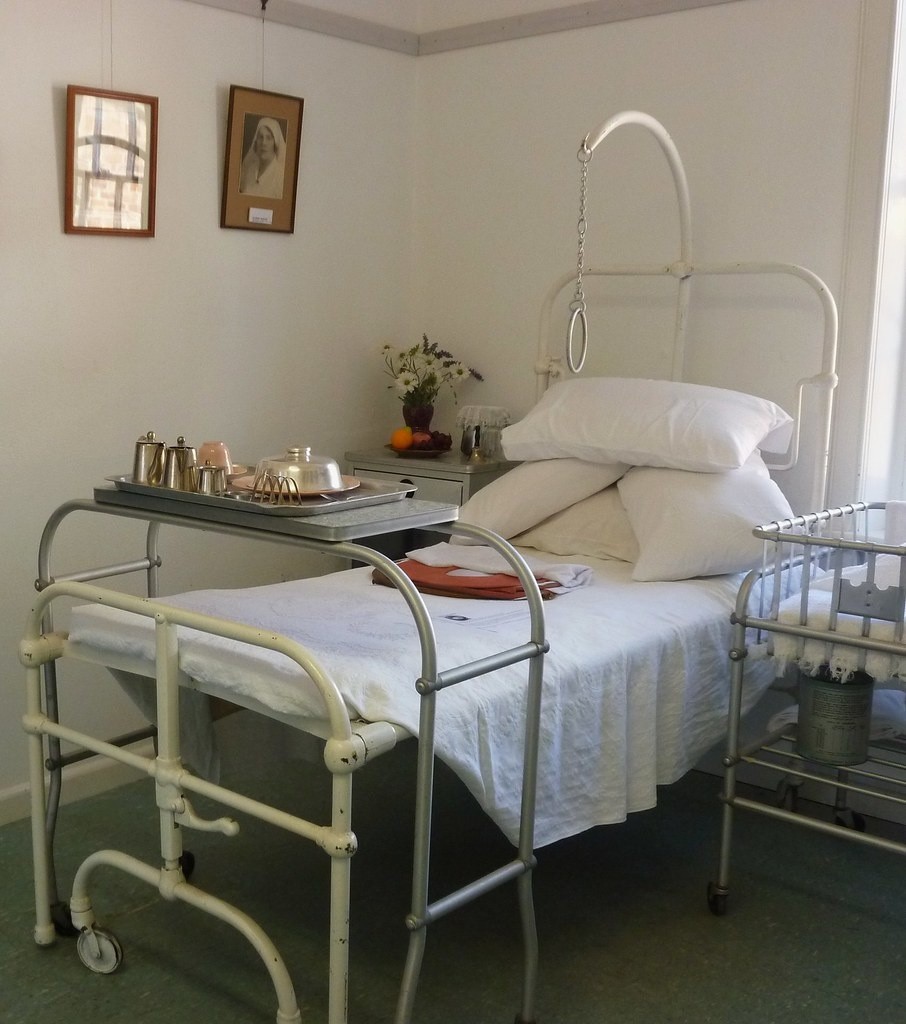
[241, 117, 287, 198]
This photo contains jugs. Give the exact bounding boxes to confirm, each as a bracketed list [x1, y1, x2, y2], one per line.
[132, 431, 197, 496]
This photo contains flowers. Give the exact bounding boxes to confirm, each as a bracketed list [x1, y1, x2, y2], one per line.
[377, 330, 485, 405]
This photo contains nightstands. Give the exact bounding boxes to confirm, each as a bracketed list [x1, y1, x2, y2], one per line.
[344, 447, 523, 570]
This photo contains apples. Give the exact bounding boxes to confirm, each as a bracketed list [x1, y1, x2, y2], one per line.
[412, 434, 430, 443]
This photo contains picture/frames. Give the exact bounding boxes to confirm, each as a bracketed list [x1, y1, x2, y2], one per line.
[64, 85, 158, 237]
[220, 85, 305, 233]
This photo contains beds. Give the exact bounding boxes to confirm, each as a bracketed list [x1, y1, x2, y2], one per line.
[19, 109, 842, 1024]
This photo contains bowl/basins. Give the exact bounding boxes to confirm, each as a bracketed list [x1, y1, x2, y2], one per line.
[196, 441, 234, 475]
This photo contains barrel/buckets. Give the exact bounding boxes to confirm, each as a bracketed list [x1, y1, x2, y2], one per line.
[797, 667, 875, 768]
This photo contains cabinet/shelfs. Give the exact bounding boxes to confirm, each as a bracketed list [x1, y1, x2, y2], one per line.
[706, 500, 906, 916]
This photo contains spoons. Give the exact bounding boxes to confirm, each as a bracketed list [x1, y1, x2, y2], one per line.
[319, 493, 379, 501]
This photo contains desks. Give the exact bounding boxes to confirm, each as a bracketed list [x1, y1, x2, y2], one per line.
[34, 486, 550, 1024]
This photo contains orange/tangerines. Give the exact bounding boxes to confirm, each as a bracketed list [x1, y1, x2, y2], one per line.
[392, 430, 413, 448]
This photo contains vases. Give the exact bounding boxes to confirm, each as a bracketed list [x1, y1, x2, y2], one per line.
[402, 402, 434, 432]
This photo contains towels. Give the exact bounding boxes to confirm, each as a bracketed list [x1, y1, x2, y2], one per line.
[883, 500, 906, 546]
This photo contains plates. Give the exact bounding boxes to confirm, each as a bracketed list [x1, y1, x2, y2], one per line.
[233, 475, 362, 494]
[384, 443, 453, 459]
[226, 465, 248, 478]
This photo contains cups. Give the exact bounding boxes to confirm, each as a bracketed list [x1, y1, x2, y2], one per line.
[195, 460, 227, 495]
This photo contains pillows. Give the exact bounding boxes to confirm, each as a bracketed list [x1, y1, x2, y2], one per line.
[507, 483, 639, 564]
[501, 378, 794, 472]
[617, 448, 804, 582]
[450, 459, 633, 544]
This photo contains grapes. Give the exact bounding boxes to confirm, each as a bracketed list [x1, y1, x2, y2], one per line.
[419, 431, 452, 450]
